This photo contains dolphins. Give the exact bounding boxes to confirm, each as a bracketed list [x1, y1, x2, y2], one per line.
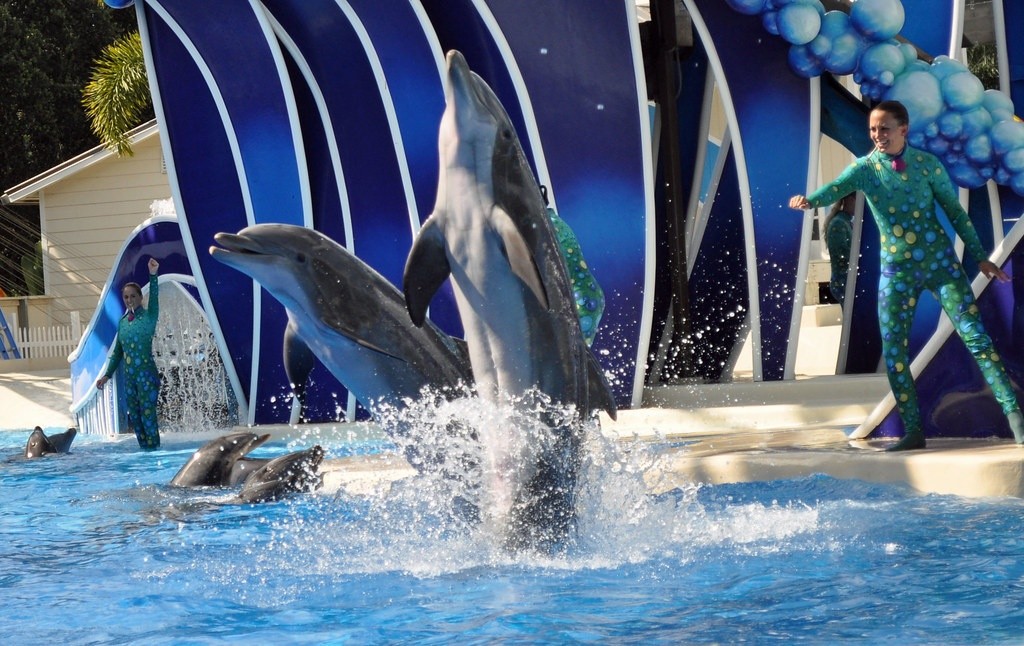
[405, 49, 618, 562]
[169, 433, 274, 486]
[207, 225, 480, 472]
[231, 446, 326, 504]
[20, 426, 78, 460]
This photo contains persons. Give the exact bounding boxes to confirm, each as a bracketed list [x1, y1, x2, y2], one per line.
[96, 257, 161, 450]
[823, 191, 856, 315]
[539, 184, 605, 347]
[788, 99, 1024, 452]
